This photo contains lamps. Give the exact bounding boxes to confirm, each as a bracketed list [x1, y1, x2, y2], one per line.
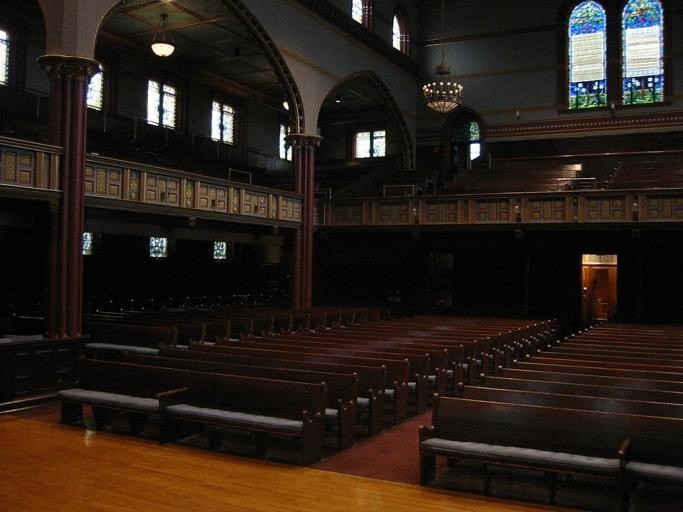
[422, 2, 464, 113]
[149, 14, 175, 57]
